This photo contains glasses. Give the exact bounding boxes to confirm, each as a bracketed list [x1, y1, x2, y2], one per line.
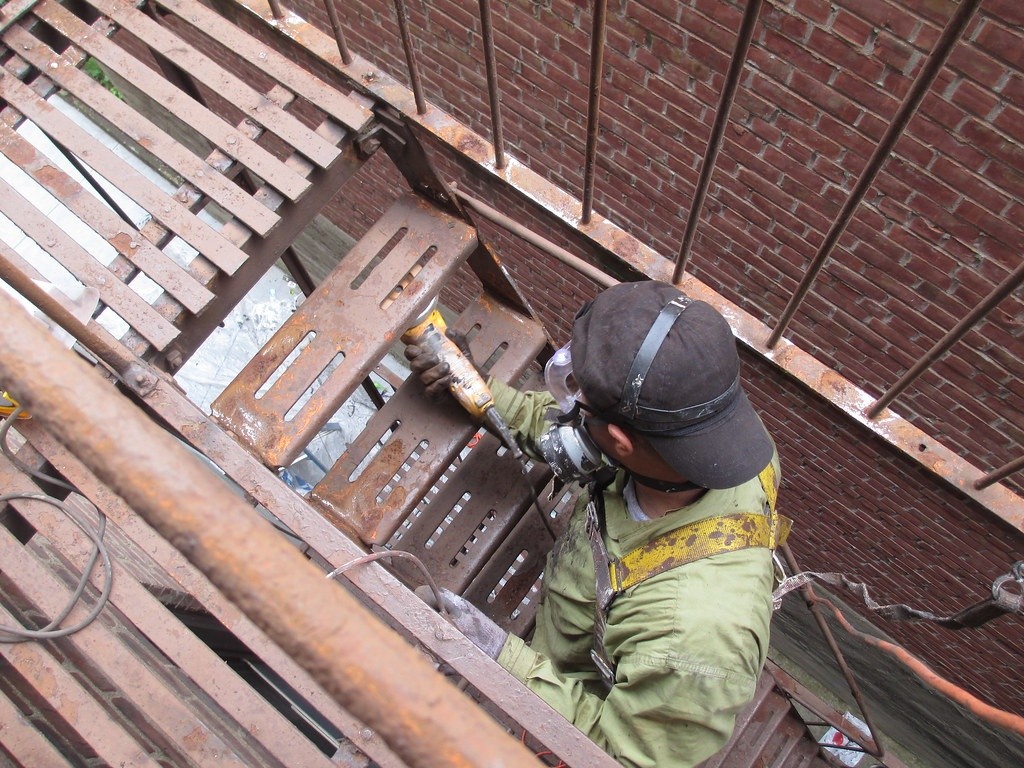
[544, 339, 597, 422]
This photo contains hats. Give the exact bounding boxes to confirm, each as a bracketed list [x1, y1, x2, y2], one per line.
[571, 280, 767, 491]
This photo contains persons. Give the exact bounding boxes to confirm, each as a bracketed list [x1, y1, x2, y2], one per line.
[404, 280, 781, 768]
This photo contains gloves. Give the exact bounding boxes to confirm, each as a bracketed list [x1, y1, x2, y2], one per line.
[404, 327, 490, 406]
[411, 585, 509, 665]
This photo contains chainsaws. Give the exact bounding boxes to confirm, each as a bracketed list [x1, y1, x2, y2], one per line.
[369, 258, 498, 414]
